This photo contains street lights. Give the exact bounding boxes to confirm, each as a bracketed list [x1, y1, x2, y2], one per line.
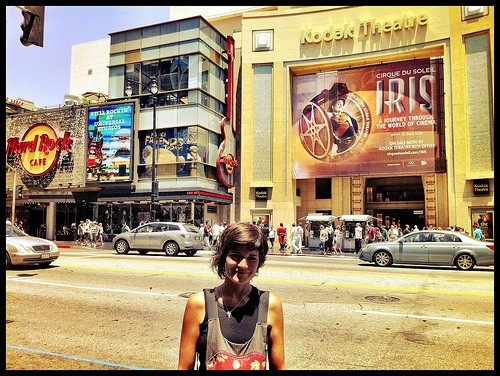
[125, 74, 162, 222]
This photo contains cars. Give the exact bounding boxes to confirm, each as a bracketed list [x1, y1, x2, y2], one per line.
[358, 230, 494, 271]
[113, 222, 204, 256]
[6, 221, 60, 270]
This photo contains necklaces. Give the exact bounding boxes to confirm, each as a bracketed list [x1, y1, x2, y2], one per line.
[219, 283, 252, 321]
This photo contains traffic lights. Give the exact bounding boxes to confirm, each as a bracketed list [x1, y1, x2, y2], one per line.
[17, 185, 23, 199]
[7, 155, 14, 164]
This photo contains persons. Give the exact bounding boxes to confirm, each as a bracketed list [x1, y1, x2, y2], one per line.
[252, 219, 303, 254]
[120, 218, 130, 234]
[139, 219, 227, 251]
[63, 219, 108, 248]
[354, 221, 482, 253]
[478, 214, 488, 239]
[320, 223, 343, 255]
[327, 96, 359, 152]
[6, 217, 46, 238]
[177, 222, 285, 370]
